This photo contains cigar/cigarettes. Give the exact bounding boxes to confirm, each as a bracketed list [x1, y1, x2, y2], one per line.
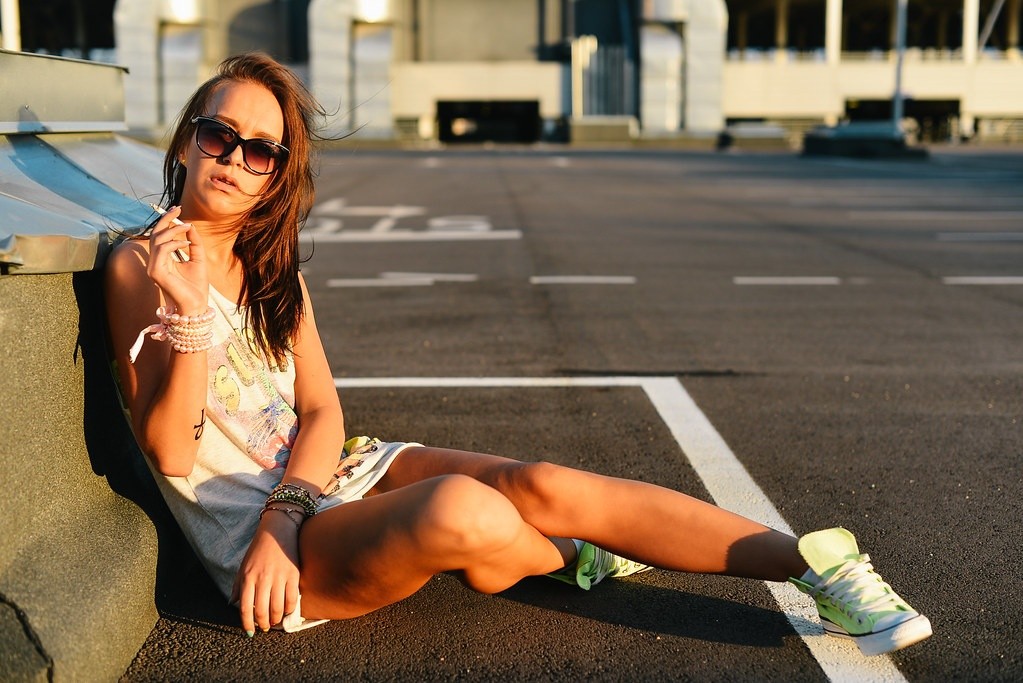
[150, 203, 186, 229]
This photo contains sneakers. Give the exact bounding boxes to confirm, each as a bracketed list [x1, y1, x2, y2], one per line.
[546, 541, 653, 589]
[788, 527, 934, 656]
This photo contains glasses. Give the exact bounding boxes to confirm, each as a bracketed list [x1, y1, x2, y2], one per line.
[190, 117, 290, 176]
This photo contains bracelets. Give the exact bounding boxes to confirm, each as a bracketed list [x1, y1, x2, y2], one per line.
[261, 484, 316, 528]
[127, 303, 215, 366]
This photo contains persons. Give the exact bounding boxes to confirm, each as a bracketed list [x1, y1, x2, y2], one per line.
[106, 53, 934, 656]
